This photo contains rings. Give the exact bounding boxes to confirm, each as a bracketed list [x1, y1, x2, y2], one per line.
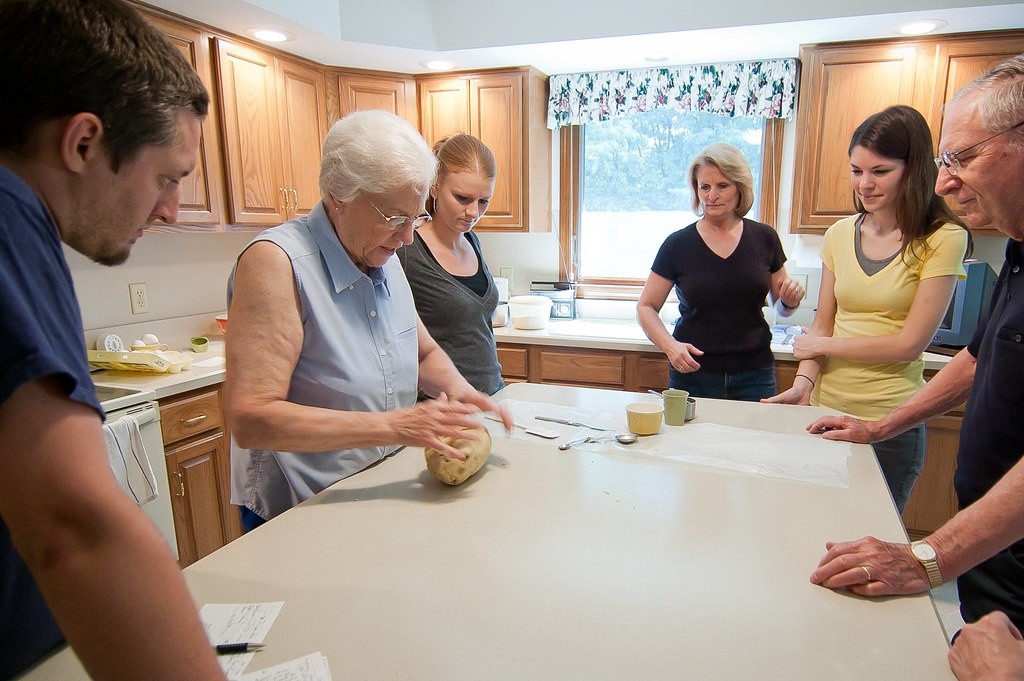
[679, 366, 683, 370]
[861, 566, 871, 582]
[795, 286, 801, 293]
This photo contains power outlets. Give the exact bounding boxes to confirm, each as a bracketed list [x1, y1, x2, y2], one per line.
[130, 283, 148, 315]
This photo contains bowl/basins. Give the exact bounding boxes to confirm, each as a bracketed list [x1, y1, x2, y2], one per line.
[625, 402, 665, 435]
[509, 296, 554, 330]
[685, 398, 696, 420]
[215, 314, 227, 334]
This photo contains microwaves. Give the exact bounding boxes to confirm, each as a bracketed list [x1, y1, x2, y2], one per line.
[931, 259, 999, 346]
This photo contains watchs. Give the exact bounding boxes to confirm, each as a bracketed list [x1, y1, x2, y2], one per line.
[910, 540, 944, 589]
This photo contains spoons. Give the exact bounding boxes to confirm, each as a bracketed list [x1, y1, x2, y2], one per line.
[558, 433, 638, 450]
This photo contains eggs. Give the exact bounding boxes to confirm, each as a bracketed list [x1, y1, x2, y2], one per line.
[120, 334, 167, 359]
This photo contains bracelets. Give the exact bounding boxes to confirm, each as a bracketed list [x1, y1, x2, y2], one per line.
[793, 374, 815, 389]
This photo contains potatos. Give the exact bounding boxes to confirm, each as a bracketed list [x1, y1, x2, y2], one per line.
[425, 424, 492, 485]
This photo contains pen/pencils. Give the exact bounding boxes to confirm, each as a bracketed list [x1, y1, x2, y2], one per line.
[211, 643, 267, 656]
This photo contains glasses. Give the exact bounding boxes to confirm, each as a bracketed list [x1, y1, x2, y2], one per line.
[360, 188, 433, 229]
[933, 121, 1024, 177]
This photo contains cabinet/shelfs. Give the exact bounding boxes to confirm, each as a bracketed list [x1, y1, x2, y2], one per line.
[491, 344, 972, 538]
[159, 387, 243, 573]
[415, 64, 554, 237]
[326, 65, 423, 217]
[788, 28, 1024, 239]
[128, 0, 222, 229]
[212, 26, 327, 226]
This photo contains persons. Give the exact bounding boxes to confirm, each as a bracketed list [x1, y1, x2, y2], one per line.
[220, 108, 513, 535]
[807, 54, 1024, 681]
[758, 104, 974, 516]
[635, 142, 805, 403]
[0, 0, 232, 681]
[395, 133, 506, 402]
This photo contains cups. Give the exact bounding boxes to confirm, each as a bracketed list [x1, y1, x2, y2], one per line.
[662, 389, 689, 427]
[131, 343, 169, 352]
[490, 276, 509, 327]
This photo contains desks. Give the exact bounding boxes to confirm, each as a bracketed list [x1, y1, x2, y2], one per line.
[13, 382, 959, 681]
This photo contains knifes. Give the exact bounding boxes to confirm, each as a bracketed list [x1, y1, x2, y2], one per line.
[535, 415, 616, 431]
[485, 415, 560, 439]
